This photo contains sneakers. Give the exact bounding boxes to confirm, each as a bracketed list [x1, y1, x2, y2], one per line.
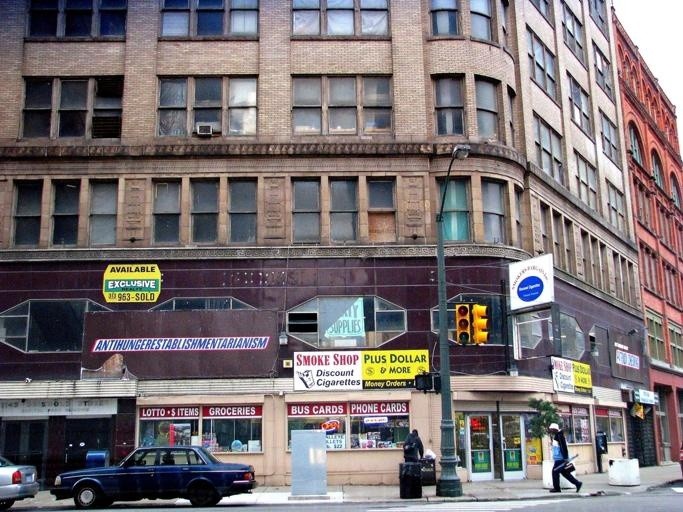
[550, 482, 582, 493]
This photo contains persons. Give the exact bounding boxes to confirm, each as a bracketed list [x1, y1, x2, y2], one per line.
[403, 429, 424, 462]
[548, 423, 582, 492]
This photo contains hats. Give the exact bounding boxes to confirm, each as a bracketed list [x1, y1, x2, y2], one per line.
[549, 423, 559, 430]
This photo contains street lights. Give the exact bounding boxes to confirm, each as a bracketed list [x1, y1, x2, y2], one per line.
[435, 144, 470, 497]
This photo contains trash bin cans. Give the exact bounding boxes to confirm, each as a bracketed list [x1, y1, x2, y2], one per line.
[419, 457, 436, 486]
[399, 462, 422, 499]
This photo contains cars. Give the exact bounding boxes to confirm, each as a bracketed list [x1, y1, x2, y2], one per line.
[0, 456, 39, 512]
[50, 445, 256, 509]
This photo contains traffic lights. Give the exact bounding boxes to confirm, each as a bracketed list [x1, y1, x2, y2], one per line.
[455, 304, 471, 344]
[414, 372, 433, 393]
[472, 304, 490, 344]
